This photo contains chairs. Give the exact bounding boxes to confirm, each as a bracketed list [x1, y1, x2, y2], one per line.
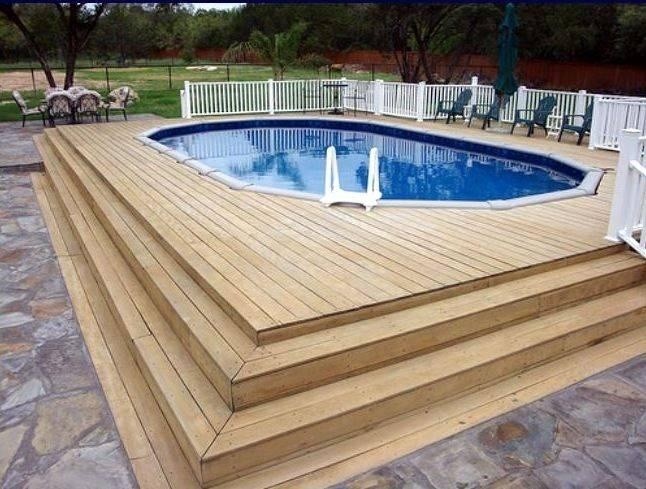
[434, 89, 595, 145]
[11, 85, 130, 127]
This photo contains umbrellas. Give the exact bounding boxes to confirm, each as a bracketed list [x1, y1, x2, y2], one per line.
[492, 3, 521, 124]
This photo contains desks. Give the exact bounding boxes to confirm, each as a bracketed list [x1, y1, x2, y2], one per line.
[322, 84, 349, 117]
